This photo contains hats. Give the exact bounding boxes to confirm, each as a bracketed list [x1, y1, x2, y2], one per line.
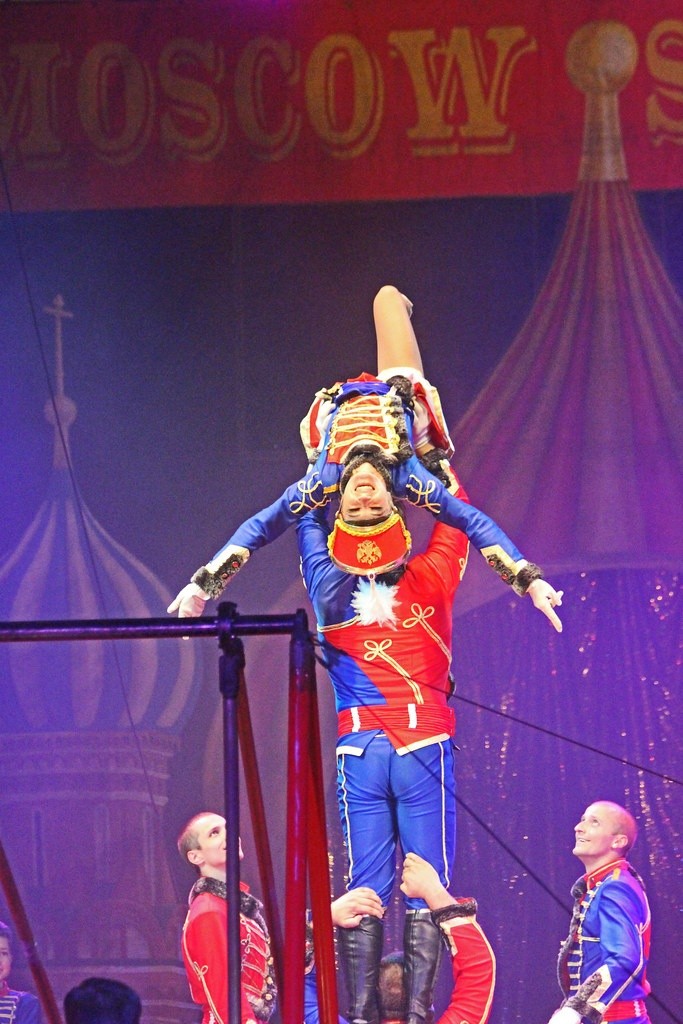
[326, 514, 412, 627]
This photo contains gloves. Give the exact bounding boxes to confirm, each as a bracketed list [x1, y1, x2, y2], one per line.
[167, 582, 211, 641]
[526, 579, 564, 633]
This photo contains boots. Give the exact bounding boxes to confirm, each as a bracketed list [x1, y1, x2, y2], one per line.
[337, 914, 380, 1024]
[404, 908, 436, 1024]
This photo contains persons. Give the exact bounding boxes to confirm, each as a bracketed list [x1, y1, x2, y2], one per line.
[167, 285, 564, 640]
[304, 852, 496, 1024]
[547, 800, 652, 1024]
[0, 921, 42, 1024]
[295, 399, 472, 1024]
[63, 976, 142, 1024]
[177, 812, 282, 1024]
